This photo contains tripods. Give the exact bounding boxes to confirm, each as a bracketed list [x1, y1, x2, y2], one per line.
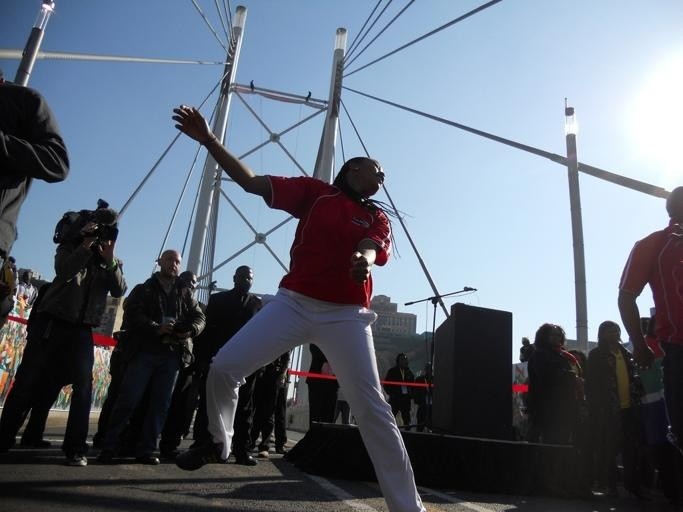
[398, 304, 458, 436]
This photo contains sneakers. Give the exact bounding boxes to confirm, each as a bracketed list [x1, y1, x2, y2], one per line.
[20, 437, 51, 447]
[256, 446, 288, 458]
[96, 449, 112, 462]
[175, 435, 222, 472]
[70, 452, 88, 467]
[140, 455, 159, 465]
[237, 456, 258, 466]
[605, 486, 617, 497]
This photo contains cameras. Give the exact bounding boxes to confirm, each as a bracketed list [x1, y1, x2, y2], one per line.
[174, 319, 193, 331]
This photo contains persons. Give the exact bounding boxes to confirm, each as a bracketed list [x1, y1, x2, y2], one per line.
[0, 76, 70, 340]
[172, 104, 429, 511]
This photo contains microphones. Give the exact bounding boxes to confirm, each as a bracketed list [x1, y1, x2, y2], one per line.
[464, 287, 476, 291]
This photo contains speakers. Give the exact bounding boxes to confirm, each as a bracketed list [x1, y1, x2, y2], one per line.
[431, 303, 512, 441]
